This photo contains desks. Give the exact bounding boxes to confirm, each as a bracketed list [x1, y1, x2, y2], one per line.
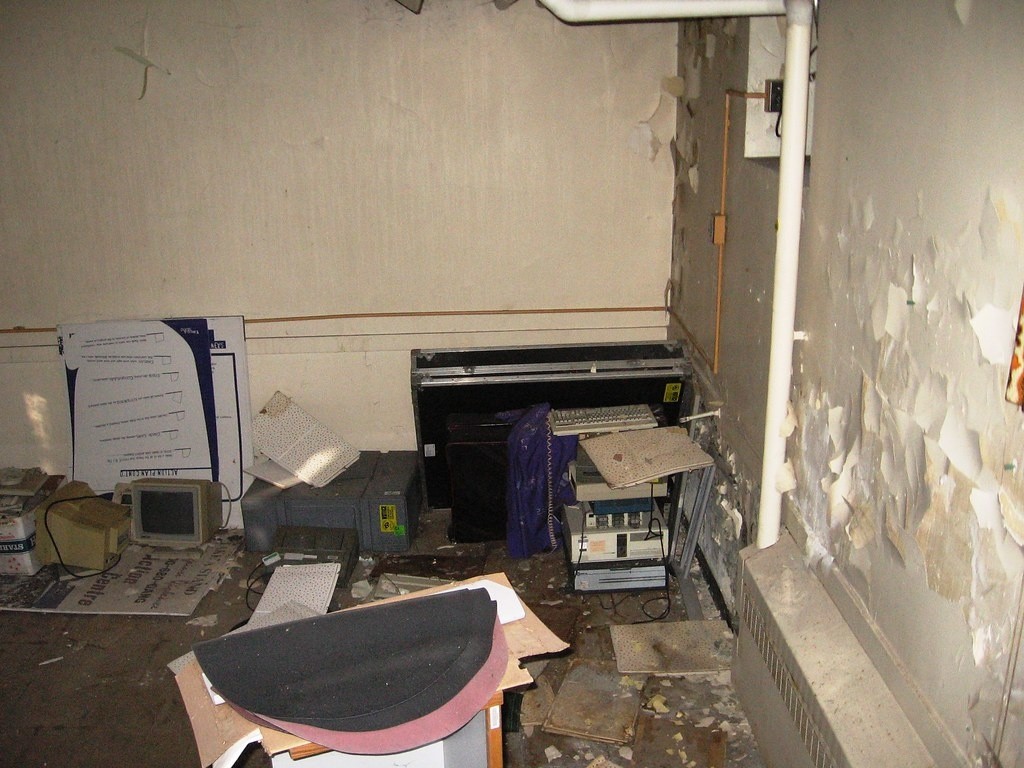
[269, 690, 503, 768]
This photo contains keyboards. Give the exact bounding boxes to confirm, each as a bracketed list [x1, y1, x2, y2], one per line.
[551, 404, 658, 436]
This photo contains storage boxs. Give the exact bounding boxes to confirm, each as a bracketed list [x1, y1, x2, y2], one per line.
[411, 339, 690, 543]
[239, 451, 419, 553]
[0, 474, 66, 576]
[446, 409, 674, 543]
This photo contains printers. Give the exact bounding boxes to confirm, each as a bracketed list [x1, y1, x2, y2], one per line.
[239, 449, 424, 589]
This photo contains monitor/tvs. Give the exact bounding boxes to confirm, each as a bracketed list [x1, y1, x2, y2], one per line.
[35, 480, 131, 571]
[130, 478, 224, 545]
